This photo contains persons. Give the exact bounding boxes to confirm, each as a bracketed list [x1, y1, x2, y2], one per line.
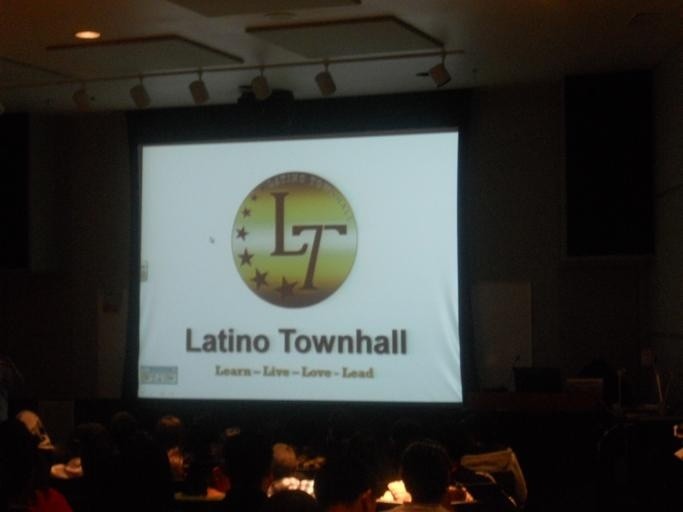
[2, 410, 527, 510]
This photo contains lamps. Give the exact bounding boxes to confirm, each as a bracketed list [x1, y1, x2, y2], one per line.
[71, 52, 453, 112]
